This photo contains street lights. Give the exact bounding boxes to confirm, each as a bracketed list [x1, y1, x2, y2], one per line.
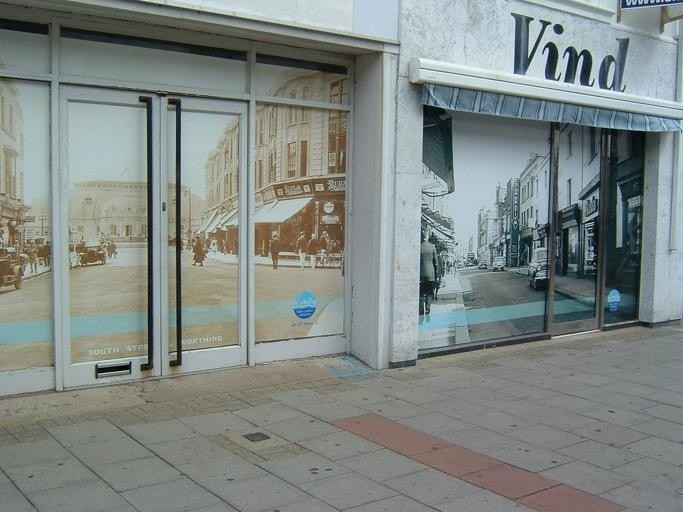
[38, 212, 47, 236]
[9, 218, 17, 244]
[183, 186, 192, 250]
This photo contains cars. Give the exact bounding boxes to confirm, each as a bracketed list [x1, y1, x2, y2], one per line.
[479, 263, 487, 269]
[584, 257, 595, 279]
[528, 247, 548, 291]
[493, 257, 506, 272]
[80, 242, 107, 267]
[0, 250, 24, 292]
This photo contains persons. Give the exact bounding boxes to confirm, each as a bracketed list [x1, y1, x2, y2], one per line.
[1, 231, 117, 273]
[418, 229, 456, 325]
[128, 233, 134, 248]
[168, 230, 330, 272]
